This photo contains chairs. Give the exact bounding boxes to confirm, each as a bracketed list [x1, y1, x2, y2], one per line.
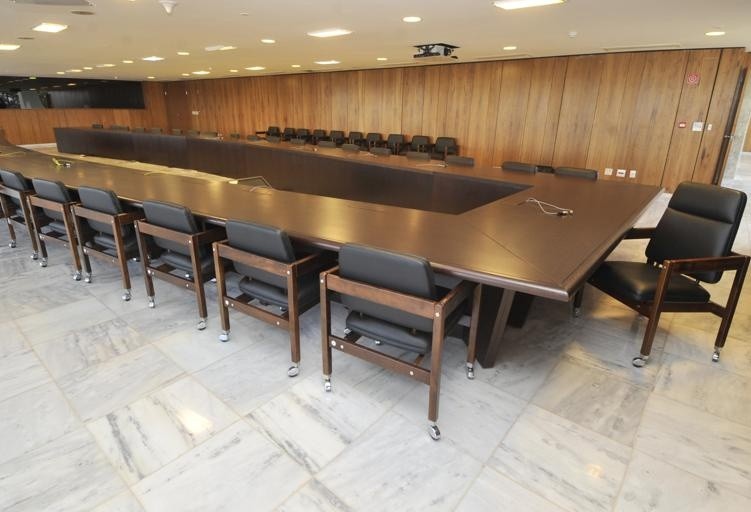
[92, 124, 599, 180]
[133, 199, 236, 331]
[69, 185, 152, 302]
[212, 218, 332, 377]
[572, 181, 749, 369]
[0, 167, 45, 260]
[26, 177, 92, 281]
[320, 241, 484, 441]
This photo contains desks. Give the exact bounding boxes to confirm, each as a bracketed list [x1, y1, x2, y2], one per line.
[1, 124, 664, 367]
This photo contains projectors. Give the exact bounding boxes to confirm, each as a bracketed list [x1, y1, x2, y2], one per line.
[418, 45, 452, 57]
